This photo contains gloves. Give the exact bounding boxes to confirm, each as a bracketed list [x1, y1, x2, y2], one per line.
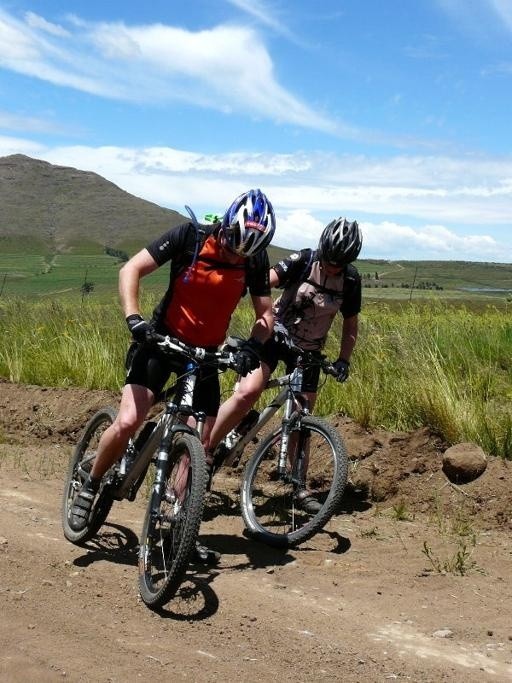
[126, 314, 153, 342]
[328, 358, 349, 382]
[237, 336, 265, 378]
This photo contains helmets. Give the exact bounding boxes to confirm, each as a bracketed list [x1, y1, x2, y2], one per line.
[222, 189, 276, 258]
[320, 217, 363, 267]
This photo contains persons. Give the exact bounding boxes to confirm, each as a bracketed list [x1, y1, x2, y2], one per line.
[205, 214, 364, 515]
[65, 185, 274, 566]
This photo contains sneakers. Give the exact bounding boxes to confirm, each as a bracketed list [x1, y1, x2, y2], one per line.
[68, 486, 95, 530]
[288, 491, 323, 513]
[193, 540, 220, 565]
